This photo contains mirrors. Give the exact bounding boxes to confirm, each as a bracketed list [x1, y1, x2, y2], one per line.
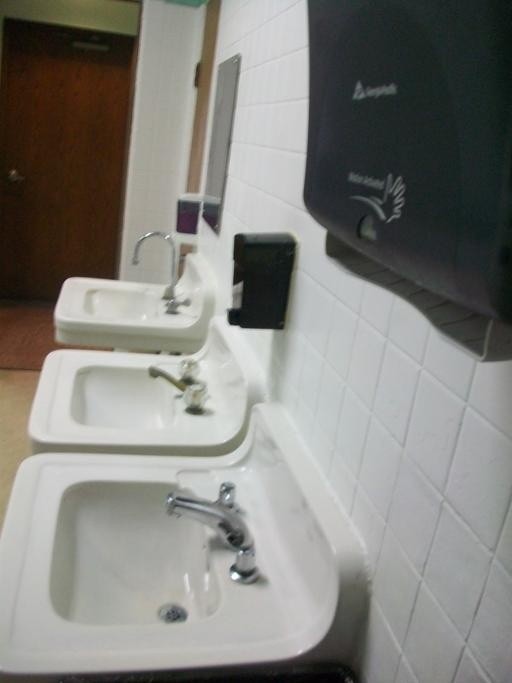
[202, 54, 240, 234]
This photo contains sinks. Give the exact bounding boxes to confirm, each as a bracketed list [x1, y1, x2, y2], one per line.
[1, 403, 366, 678]
[27, 317, 265, 451]
[53, 252, 216, 345]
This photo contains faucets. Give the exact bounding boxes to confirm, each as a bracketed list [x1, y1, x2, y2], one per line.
[148, 361, 207, 413]
[131, 230, 180, 300]
[165, 492, 257, 551]
